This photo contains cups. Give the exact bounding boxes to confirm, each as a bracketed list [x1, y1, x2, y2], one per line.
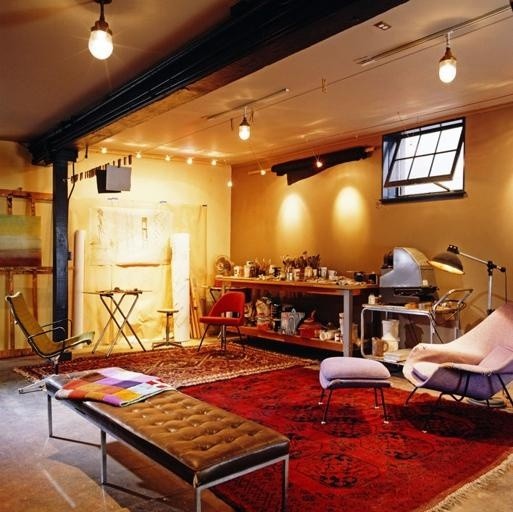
[371, 338, 389, 356]
[327, 270, 338, 278]
[317, 267, 327, 278]
[382, 319, 399, 338]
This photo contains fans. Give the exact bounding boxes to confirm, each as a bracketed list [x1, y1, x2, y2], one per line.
[213, 255, 236, 277]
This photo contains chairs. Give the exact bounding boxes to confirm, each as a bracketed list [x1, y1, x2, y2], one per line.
[5, 292, 95, 393]
[192, 291, 247, 355]
[401, 298, 512, 436]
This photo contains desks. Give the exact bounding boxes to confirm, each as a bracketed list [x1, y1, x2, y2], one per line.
[208, 287, 252, 341]
[82, 288, 152, 359]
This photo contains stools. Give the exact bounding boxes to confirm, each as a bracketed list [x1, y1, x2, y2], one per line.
[151, 306, 186, 350]
[316, 355, 394, 426]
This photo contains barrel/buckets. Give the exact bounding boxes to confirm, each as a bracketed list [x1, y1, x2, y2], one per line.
[381, 318, 401, 338]
[383, 336, 400, 353]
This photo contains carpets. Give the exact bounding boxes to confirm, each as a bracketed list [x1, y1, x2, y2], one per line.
[10, 339, 322, 398]
[175, 365, 513, 510]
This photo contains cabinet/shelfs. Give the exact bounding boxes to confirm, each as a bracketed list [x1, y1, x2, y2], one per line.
[214, 273, 379, 357]
[358, 289, 471, 367]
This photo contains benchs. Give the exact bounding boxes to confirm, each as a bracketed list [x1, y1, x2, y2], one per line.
[42, 364, 292, 510]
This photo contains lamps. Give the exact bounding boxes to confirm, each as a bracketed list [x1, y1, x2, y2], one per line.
[84, 0, 116, 61]
[438, 30, 457, 84]
[236, 106, 250, 141]
[428, 244, 506, 315]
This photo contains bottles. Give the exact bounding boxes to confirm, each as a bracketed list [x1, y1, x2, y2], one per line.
[339, 313, 343, 337]
[355, 272, 363, 282]
[367, 292, 376, 304]
[232, 262, 315, 283]
[335, 334, 339, 341]
